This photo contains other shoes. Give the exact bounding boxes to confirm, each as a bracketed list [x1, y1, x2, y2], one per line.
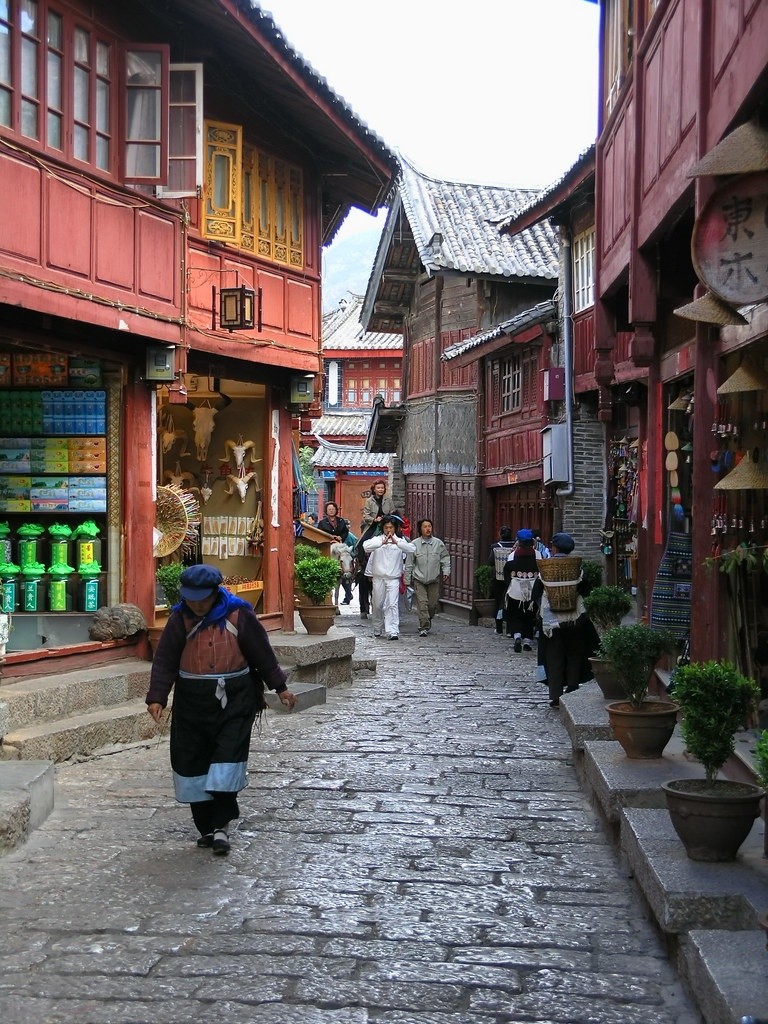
[196, 834, 213, 847]
[514, 638, 522, 652]
[349, 594, 353, 600]
[340, 601, 348, 605]
[494, 629, 503, 635]
[506, 633, 511, 637]
[550, 699, 559, 707]
[360, 612, 367, 619]
[213, 830, 230, 853]
[523, 642, 532, 650]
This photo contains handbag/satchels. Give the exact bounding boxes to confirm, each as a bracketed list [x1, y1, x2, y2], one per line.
[404, 586, 414, 612]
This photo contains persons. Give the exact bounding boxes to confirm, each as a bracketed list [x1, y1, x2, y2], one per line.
[489, 526, 603, 709]
[362, 516, 416, 640]
[403, 519, 451, 636]
[144, 565, 295, 854]
[306, 480, 411, 619]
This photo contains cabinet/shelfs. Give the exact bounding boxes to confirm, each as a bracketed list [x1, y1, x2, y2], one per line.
[0, 386, 111, 616]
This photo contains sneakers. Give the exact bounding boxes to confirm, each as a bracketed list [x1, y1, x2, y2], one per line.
[417, 626, 422, 631]
[388, 633, 399, 640]
[374, 631, 382, 637]
[420, 630, 428, 636]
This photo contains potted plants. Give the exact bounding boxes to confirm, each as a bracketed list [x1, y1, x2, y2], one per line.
[474, 565, 497, 618]
[597, 623, 680, 759]
[661, 657, 766, 862]
[582, 584, 634, 700]
[147, 560, 184, 659]
[294, 544, 342, 635]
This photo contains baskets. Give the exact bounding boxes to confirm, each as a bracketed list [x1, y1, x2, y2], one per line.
[494, 543, 514, 580]
[537, 557, 582, 612]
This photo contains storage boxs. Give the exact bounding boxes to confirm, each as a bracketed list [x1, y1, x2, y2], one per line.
[0, 355, 107, 512]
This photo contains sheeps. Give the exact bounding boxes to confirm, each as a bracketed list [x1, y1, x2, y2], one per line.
[224, 472, 262, 503]
[219, 440, 263, 469]
[164, 470, 194, 490]
[172, 392, 232, 462]
[160, 430, 190, 459]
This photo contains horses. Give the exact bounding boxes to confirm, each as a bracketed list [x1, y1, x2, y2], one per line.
[330, 543, 354, 615]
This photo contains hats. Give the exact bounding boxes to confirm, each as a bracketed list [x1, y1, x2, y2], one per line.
[517, 529, 533, 540]
[500, 526, 513, 539]
[180, 564, 222, 602]
[553, 533, 575, 553]
